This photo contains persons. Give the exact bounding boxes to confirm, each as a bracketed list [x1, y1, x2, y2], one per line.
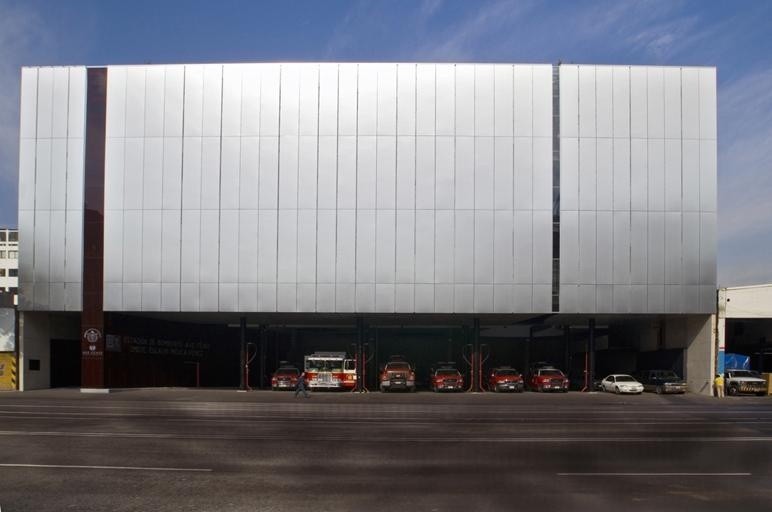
[714, 374, 725, 399]
[294, 372, 308, 399]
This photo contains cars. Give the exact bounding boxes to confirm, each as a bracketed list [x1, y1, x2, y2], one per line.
[271, 365, 301, 390]
[599, 368, 689, 394]
[724, 368, 768, 396]
[378, 351, 570, 394]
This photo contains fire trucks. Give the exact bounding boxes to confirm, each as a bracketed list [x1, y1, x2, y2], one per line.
[303, 352, 357, 392]
